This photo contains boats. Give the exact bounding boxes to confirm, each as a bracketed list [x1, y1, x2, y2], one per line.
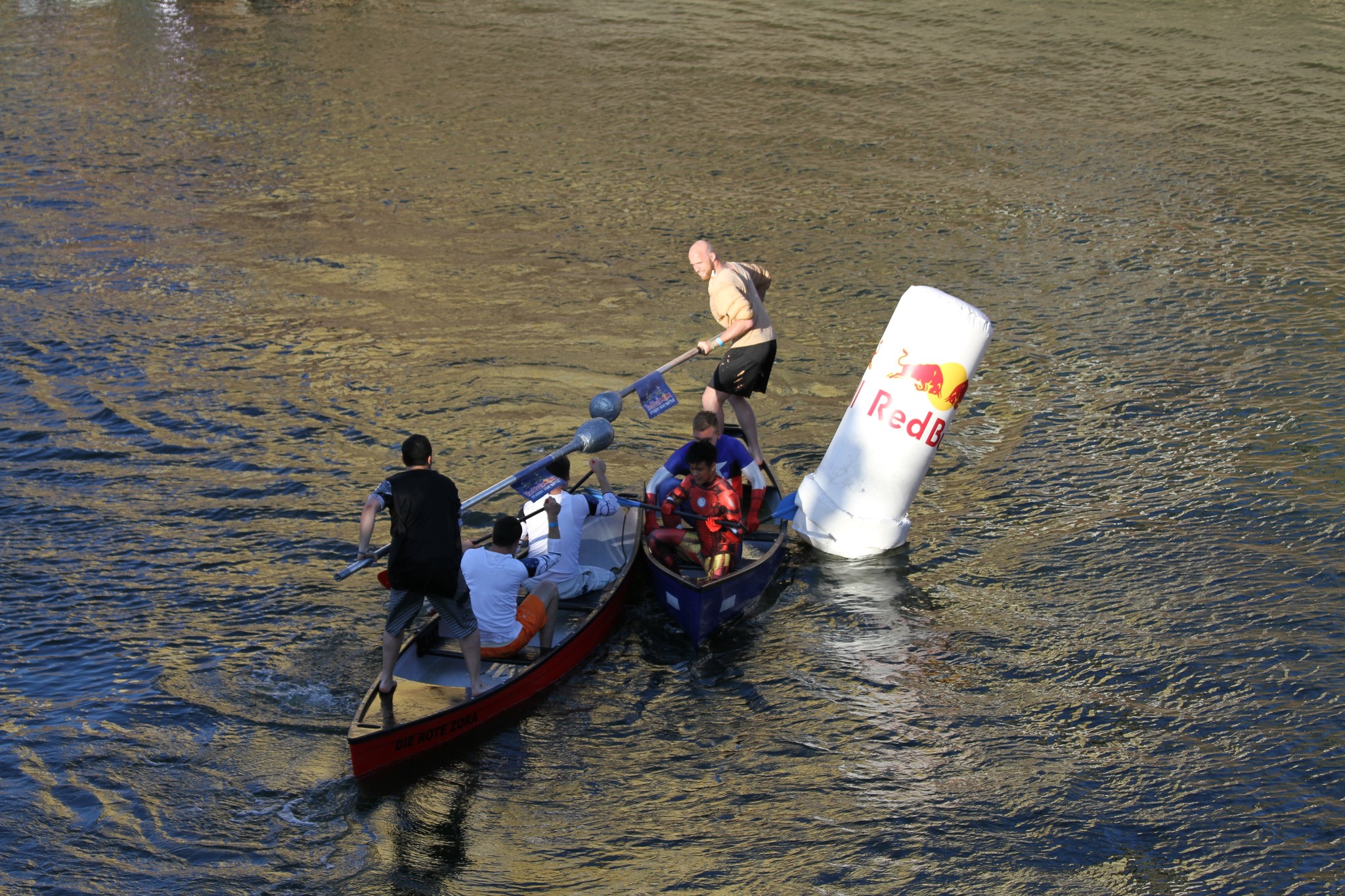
[641, 421, 790, 630]
[343, 480, 647, 778]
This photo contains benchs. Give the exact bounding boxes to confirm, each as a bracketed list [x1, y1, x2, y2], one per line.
[642, 524, 781, 574]
[416, 584, 610, 666]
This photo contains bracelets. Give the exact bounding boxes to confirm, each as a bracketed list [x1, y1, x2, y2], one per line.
[711, 341, 715, 350]
[715, 337, 723, 347]
[358, 550, 370, 556]
[549, 521, 558, 527]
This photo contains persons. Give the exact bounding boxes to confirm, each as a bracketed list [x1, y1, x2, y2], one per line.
[688, 240, 777, 465]
[358, 434, 494, 698]
[515, 452, 620, 597]
[460, 496, 562, 659]
[645, 410, 767, 585]
